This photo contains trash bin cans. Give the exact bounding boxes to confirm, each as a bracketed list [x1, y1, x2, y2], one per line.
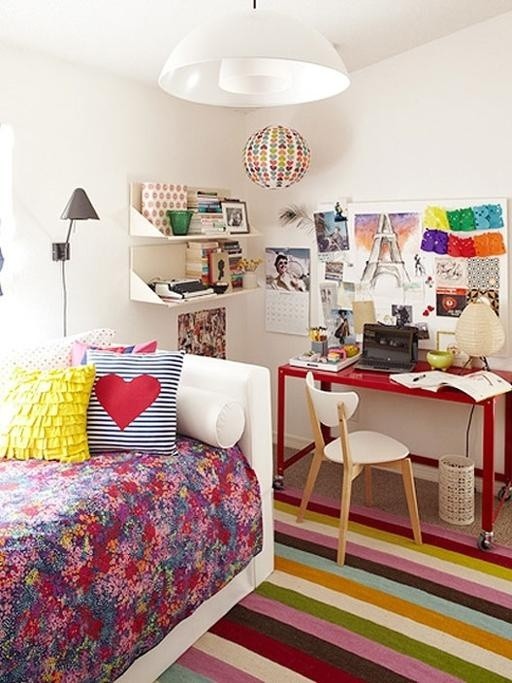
[438, 453, 475, 526]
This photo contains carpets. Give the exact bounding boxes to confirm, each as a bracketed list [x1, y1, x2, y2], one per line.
[160, 492, 512, 682]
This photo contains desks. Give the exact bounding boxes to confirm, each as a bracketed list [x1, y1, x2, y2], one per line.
[270, 348, 511, 557]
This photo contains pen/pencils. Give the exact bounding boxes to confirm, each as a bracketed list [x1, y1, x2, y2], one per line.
[413, 374, 425, 381]
[309, 326, 327, 342]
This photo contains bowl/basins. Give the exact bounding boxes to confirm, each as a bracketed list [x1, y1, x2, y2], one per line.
[427, 350, 453, 371]
[212, 281, 229, 293]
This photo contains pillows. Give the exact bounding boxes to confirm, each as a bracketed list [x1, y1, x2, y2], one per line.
[0, 329, 248, 464]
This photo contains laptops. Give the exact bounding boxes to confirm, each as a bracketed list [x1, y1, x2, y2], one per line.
[353, 323, 419, 374]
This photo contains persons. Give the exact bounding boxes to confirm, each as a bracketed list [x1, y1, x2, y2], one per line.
[334, 309, 350, 340]
[178, 308, 226, 358]
[328, 225, 343, 249]
[334, 202, 347, 221]
[275, 255, 300, 291]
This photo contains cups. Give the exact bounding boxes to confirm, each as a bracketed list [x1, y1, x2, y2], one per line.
[166, 209, 194, 237]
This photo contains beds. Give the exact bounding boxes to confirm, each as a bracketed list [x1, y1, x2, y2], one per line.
[0, 329, 281, 683]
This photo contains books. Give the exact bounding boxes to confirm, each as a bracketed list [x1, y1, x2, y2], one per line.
[422, 369, 512, 402]
[390, 369, 462, 388]
[184, 187, 244, 288]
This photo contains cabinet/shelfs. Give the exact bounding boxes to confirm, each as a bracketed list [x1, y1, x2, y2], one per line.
[127, 181, 263, 310]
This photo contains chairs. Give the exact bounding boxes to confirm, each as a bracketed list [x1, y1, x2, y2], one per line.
[295, 370, 425, 569]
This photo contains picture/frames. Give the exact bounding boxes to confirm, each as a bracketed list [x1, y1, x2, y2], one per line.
[218, 197, 251, 237]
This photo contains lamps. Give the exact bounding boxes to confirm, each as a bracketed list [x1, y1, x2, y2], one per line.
[451, 299, 505, 376]
[50, 186, 102, 340]
[155, 0, 354, 112]
